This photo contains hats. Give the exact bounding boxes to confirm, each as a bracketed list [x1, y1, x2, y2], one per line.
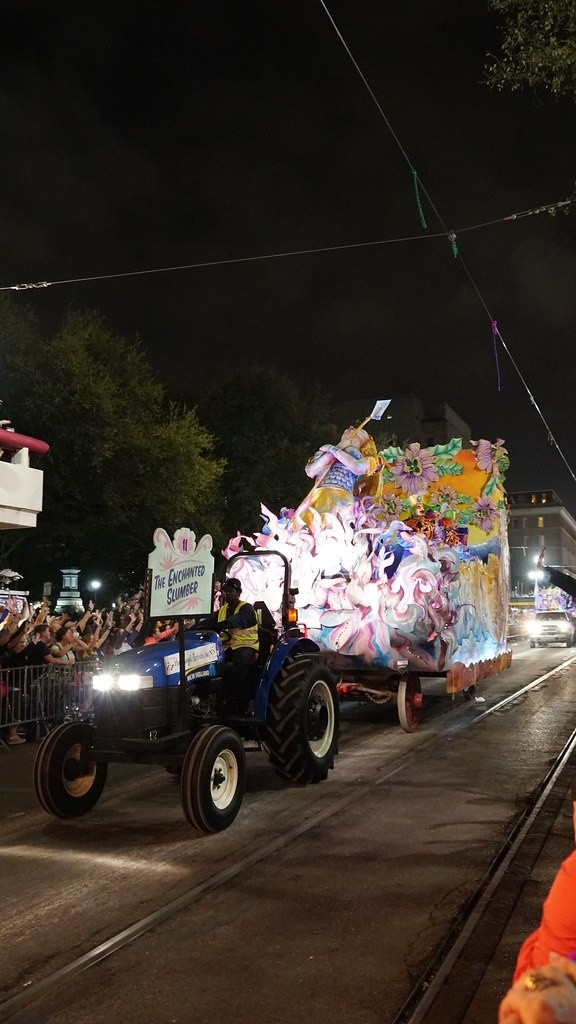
[222, 578, 242, 593]
[65, 620, 77, 627]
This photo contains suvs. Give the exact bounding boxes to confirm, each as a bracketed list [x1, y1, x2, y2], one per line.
[529, 611, 574, 647]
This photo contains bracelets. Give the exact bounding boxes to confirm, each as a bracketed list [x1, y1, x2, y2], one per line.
[10, 612, 13, 613]
[330, 447, 340, 454]
[107, 628, 111, 630]
[87, 609, 91, 611]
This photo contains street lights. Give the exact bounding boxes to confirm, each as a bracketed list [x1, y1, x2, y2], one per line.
[91, 580, 100, 608]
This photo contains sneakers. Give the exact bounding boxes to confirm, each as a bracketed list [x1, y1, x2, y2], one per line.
[7, 735, 27, 745]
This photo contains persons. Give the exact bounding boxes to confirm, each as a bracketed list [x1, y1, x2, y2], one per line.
[292, 426, 378, 525]
[0, 584, 196, 744]
[537, 547, 576, 598]
[214, 581, 221, 611]
[190, 578, 259, 715]
[498, 799, 576, 1024]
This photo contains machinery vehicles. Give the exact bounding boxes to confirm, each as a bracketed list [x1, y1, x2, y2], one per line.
[32, 550, 341, 834]
